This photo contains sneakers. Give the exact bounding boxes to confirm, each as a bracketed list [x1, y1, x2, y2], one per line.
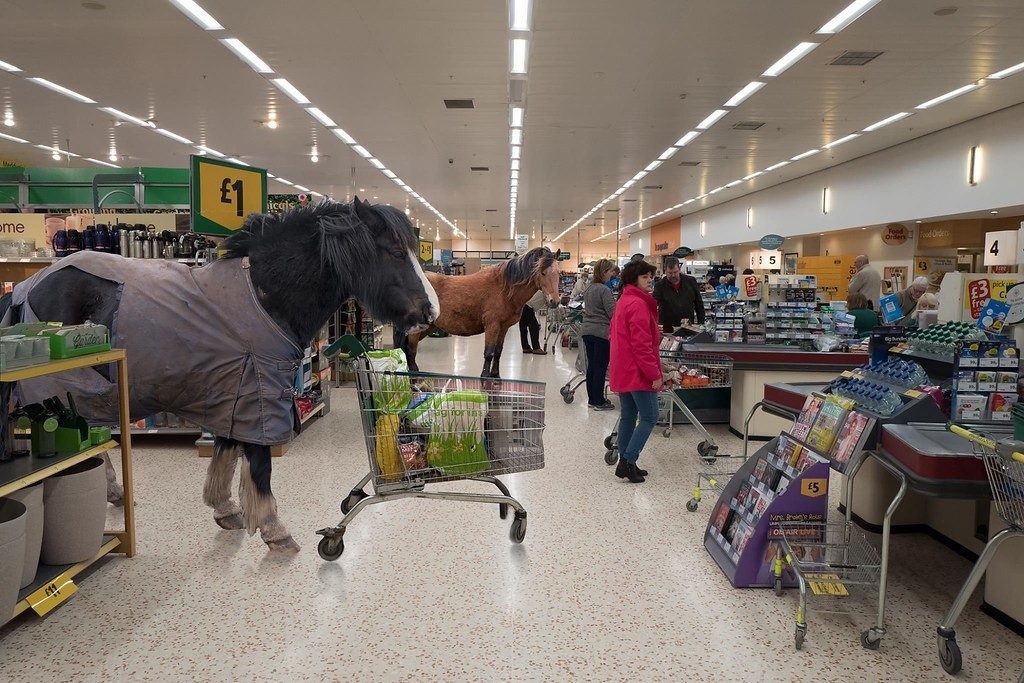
[533, 349, 547, 354]
[595, 401, 615, 410]
[523, 348, 533, 353]
[588, 399, 611, 407]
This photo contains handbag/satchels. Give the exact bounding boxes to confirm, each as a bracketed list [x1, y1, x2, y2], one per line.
[365, 345, 490, 474]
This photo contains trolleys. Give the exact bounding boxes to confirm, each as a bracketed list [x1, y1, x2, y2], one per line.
[773, 453, 906, 650]
[542, 304, 612, 404]
[316, 332, 547, 562]
[604, 351, 735, 465]
[686, 402, 796, 512]
[937, 422, 1024, 683]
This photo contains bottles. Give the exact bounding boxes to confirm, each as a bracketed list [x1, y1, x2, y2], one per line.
[859, 357, 934, 392]
[905, 320, 990, 358]
[53, 223, 215, 259]
[829, 376, 904, 417]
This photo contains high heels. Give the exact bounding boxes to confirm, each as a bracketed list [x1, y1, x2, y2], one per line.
[615, 458, 645, 483]
[636, 464, 648, 476]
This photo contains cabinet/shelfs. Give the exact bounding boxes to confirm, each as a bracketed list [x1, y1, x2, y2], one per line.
[0, 153, 383, 620]
[703, 429, 831, 588]
[693, 274, 830, 345]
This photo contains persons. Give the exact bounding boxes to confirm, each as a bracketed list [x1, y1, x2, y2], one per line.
[608, 261, 663, 483]
[847, 255, 882, 313]
[519, 289, 547, 355]
[882, 276, 929, 324]
[604, 266, 620, 288]
[582, 259, 616, 411]
[650, 257, 706, 333]
[570, 272, 591, 299]
[706, 269, 753, 290]
[903, 293, 939, 327]
[845, 293, 879, 338]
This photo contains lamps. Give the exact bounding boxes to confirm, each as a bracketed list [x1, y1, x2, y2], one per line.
[822, 188, 830, 215]
[968, 147, 979, 186]
[746, 208, 752, 228]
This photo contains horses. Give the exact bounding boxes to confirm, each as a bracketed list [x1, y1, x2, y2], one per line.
[0, 196, 440, 554]
[400, 247, 559, 391]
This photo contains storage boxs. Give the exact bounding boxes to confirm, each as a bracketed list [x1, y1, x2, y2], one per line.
[311, 319, 331, 417]
[298, 347, 312, 389]
[715, 434, 817, 556]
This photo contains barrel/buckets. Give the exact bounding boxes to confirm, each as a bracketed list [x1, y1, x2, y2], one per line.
[0, 458, 107, 627]
